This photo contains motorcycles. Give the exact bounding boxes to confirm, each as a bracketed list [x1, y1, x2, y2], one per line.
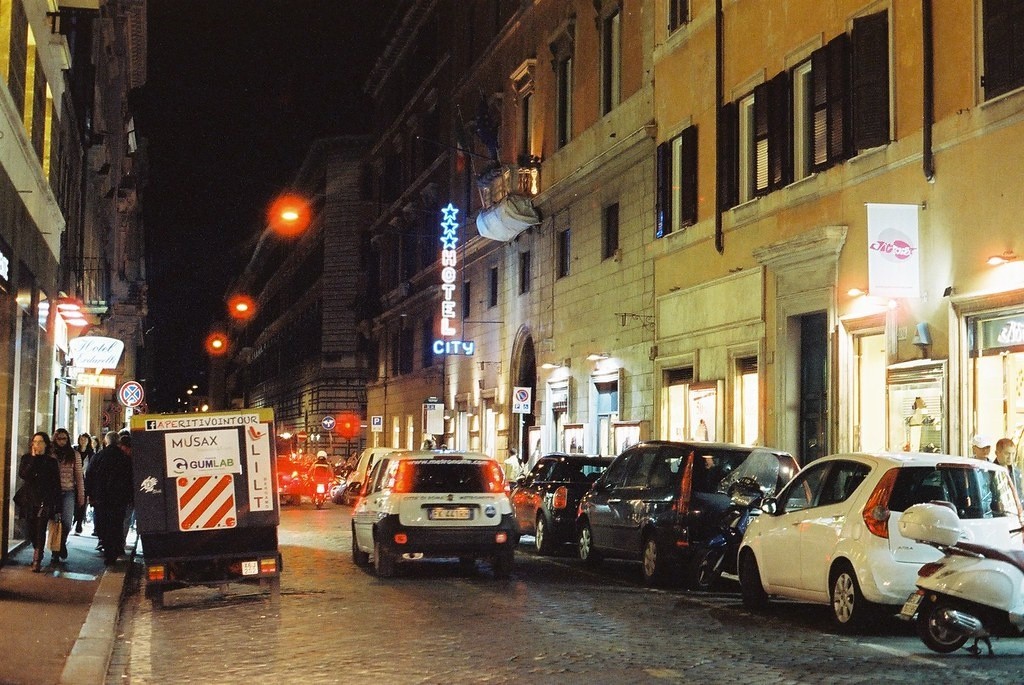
[310, 464, 330, 510]
[898, 500, 1024, 657]
[691, 449, 779, 591]
[131, 407, 283, 607]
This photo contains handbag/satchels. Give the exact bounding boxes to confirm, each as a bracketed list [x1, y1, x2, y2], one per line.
[46, 518, 62, 551]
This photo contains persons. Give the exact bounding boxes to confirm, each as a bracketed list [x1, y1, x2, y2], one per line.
[308, 450, 334, 492]
[84, 431, 132, 566]
[71, 433, 96, 533]
[47, 428, 85, 572]
[502, 448, 524, 494]
[993, 438, 1024, 527]
[11, 432, 62, 571]
[89, 436, 101, 454]
[970, 433, 992, 463]
[96, 429, 133, 560]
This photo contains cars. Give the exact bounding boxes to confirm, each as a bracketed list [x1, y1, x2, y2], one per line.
[575, 439, 813, 590]
[505, 440, 619, 557]
[735, 452, 1024, 633]
[341, 447, 520, 580]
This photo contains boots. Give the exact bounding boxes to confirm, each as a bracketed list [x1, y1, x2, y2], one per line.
[32, 539, 45, 573]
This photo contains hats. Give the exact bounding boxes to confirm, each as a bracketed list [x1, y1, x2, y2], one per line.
[972, 434, 992, 448]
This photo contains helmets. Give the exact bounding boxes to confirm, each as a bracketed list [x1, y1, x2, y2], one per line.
[317, 451, 328, 460]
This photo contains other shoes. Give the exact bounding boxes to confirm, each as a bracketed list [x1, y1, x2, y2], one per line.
[103, 558, 117, 566]
[92, 531, 97, 535]
[98, 541, 103, 550]
[76, 525, 83, 532]
[59, 543, 68, 560]
[49, 561, 60, 571]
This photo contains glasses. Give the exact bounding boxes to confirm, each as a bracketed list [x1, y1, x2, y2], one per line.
[32, 440, 46, 445]
[57, 436, 68, 441]
